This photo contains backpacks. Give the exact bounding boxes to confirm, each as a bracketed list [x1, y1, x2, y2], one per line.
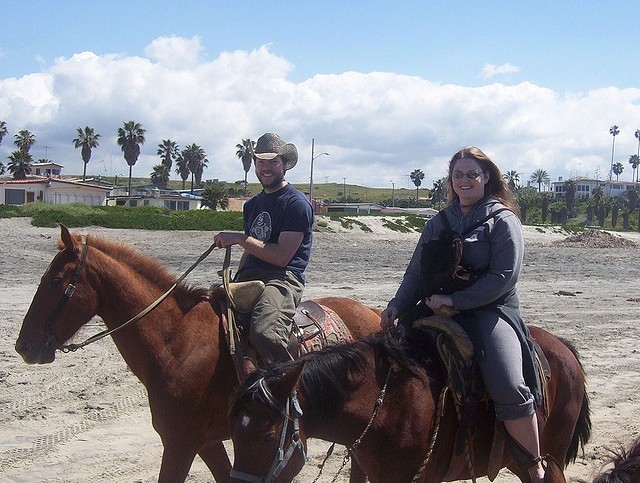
[419, 208, 514, 293]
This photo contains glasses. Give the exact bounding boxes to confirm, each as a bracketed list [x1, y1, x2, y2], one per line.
[452, 169, 485, 178]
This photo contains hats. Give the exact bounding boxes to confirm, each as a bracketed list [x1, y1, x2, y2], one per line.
[247, 132, 298, 171]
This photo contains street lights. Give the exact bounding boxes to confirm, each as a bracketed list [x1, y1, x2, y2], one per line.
[309, 139, 330, 206]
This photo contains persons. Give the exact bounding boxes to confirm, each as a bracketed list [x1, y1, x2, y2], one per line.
[379, 147, 547, 482]
[214, 133, 314, 418]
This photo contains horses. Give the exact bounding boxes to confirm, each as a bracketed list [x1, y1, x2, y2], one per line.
[14, 222, 395, 482]
[228, 323, 595, 483]
